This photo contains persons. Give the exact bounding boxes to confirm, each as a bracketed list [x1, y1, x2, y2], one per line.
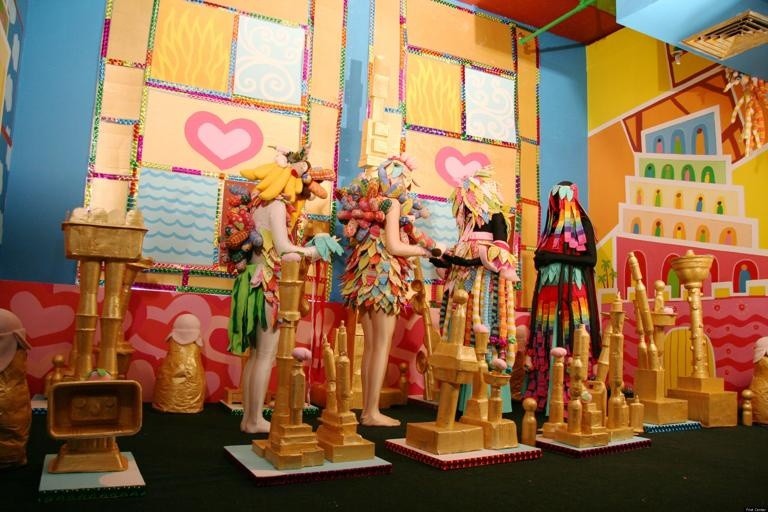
[430, 171, 518, 419]
[519, 180, 604, 421]
[214, 141, 346, 432]
[331, 153, 443, 430]
